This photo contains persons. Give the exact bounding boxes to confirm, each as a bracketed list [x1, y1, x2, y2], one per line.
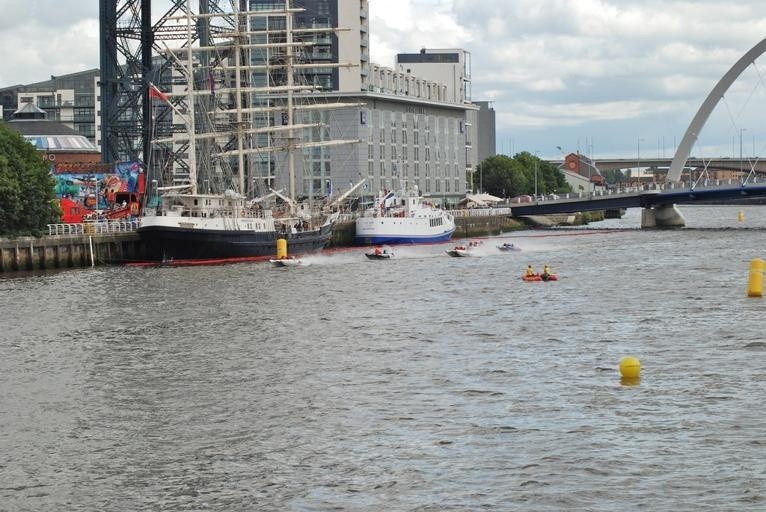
[121, 200, 127, 208]
[544, 265, 555, 275]
[526, 265, 539, 277]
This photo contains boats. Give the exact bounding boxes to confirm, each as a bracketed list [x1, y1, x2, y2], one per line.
[269, 256, 303, 266]
[521, 272, 559, 281]
[445, 238, 524, 256]
[355, 179, 456, 245]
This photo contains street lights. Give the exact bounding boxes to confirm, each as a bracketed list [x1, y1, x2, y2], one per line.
[90, 178, 98, 223]
[638, 137, 646, 182]
[740, 128, 747, 184]
[688, 133, 696, 190]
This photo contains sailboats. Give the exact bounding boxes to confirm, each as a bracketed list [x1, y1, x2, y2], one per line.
[135, 0, 365, 259]
